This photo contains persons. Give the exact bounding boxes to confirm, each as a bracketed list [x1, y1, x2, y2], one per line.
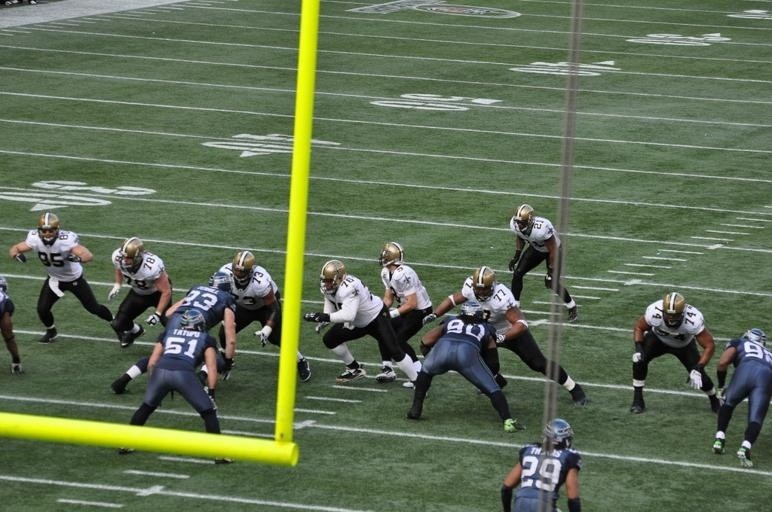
[376, 242, 433, 387]
[506, 204, 577, 323]
[108, 236, 310, 462]
[423, 266, 590, 408]
[1, 277, 23, 375]
[711, 328, 770, 468]
[501, 418, 581, 512]
[304, 260, 429, 394]
[9, 213, 121, 343]
[629, 291, 723, 413]
[407, 301, 527, 432]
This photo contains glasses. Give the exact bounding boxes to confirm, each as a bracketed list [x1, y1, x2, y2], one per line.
[41, 229, 53, 233]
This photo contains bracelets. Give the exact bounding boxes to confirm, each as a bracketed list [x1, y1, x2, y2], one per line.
[390, 310, 399, 321]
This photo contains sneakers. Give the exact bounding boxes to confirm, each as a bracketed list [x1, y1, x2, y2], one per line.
[479, 374, 507, 396]
[38, 327, 58, 344]
[736, 446, 754, 468]
[712, 438, 725, 455]
[711, 400, 720, 413]
[504, 418, 527, 433]
[109, 381, 129, 393]
[121, 323, 146, 348]
[403, 380, 415, 388]
[337, 364, 367, 384]
[567, 305, 577, 322]
[631, 400, 645, 414]
[297, 357, 311, 382]
[573, 391, 587, 407]
[376, 366, 397, 383]
[109, 319, 122, 345]
[408, 408, 421, 419]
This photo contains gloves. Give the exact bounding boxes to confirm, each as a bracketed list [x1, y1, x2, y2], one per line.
[421, 314, 437, 325]
[715, 388, 727, 408]
[316, 322, 330, 334]
[495, 333, 505, 344]
[254, 325, 273, 348]
[216, 358, 236, 382]
[11, 362, 23, 375]
[509, 251, 520, 271]
[632, 341, 644, 364]
[15, 252, 26, 263]
[66, 253, 80, 262]
[544, 266, 553, 290]
[208, 396, 218, 410]
[108, 287, 120, 302]
[145, 312, 161, 327]
[303, 312, 324, 322]
[685, 365, 703, 390]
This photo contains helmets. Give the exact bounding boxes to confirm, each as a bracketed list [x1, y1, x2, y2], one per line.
[513, 204, 534, 237]
[208, 270, 232, 295]
[472, 266, 495, 303]
[742, 327, 766, 347]
[119, 237, 144, 277]
[38, 212, 60, 249]
[320, 260, 347, 297]
[540, 419, 574, 449]
[379, 241, 403, 267]
[178, 308, 207, 332]
[459, 301, 483, 320]
[232, 251, 255, 293]
[663, 292, 685, 330]
